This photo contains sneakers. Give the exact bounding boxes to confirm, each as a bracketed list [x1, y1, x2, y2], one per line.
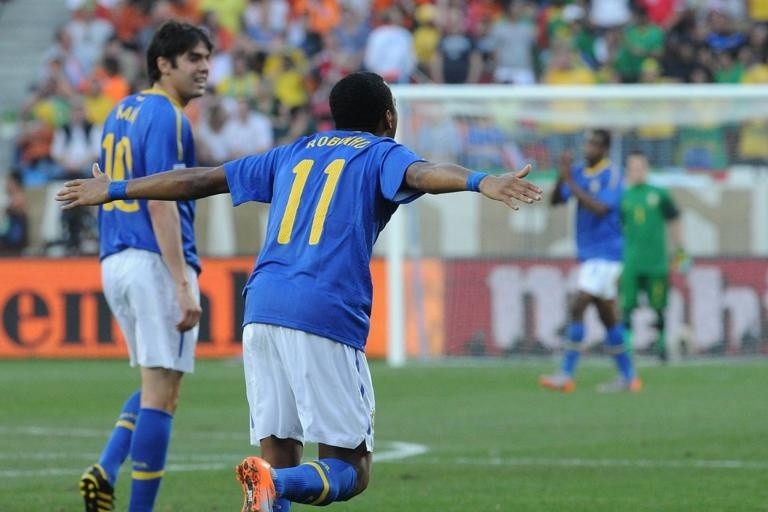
[80, 465, 114, 512]
[234, 457, 277, 512]
[538, 374, 643, 394]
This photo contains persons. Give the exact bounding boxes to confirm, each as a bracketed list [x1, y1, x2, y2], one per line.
[603, 151, 688, 367]
[78, 16, 213, 510]
[51, 67, 543, 512]
[533, 127, 644, 394]
